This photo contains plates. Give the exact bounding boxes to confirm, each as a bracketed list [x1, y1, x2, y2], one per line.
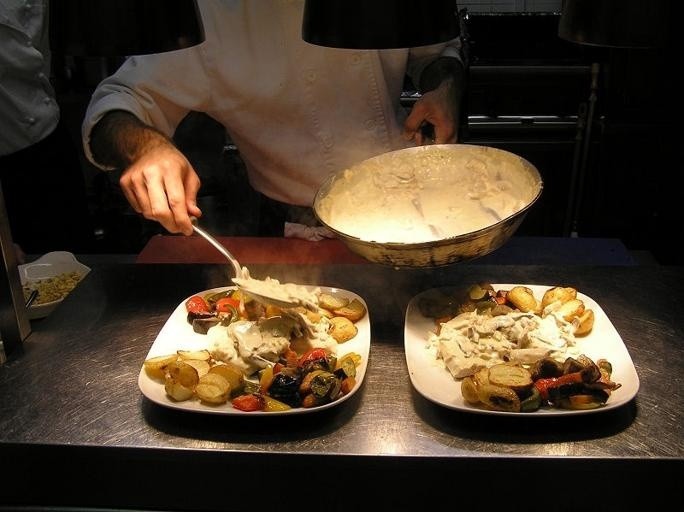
[138, 282, 373, 416]
[404, 283, 641, 416]
[17, 251, 92, 319]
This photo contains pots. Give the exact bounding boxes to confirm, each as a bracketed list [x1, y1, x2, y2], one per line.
[313, 120, 544, 270]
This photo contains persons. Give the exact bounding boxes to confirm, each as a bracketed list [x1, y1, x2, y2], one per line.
[0, 0, 102, 265]
[82, 0, 463, 284]
